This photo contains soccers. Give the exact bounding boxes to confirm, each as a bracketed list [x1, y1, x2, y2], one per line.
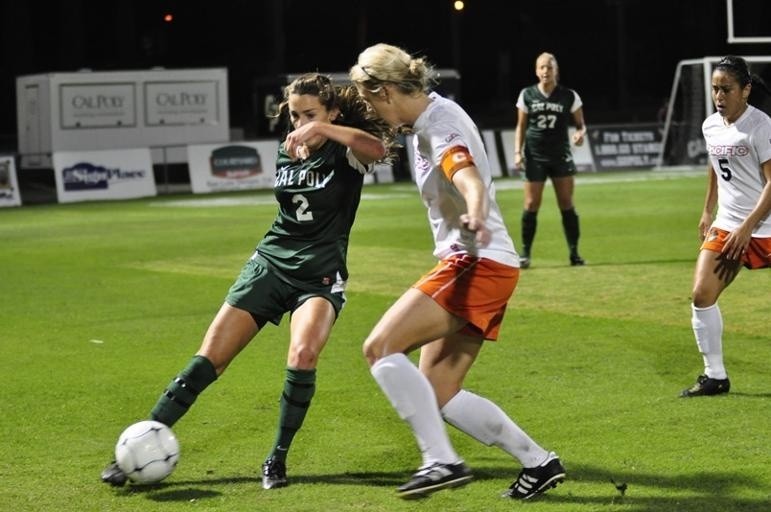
[115, 421, 179, 484]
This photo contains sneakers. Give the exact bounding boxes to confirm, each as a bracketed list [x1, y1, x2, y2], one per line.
[677, 374, 730, 399]
[394, 460, 475, 497]
[569, 254, 584, 266]
[101, 462, 128, 488]
[262, 454, 287, 490]
[500, 450, 566, 501]
[518, 256, 530, 269]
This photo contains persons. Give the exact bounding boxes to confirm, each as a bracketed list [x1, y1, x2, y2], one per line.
[101, 73, 386, 490]
[350, 43, 565, 501]
[514, 52, 587, 268]
[679, 55, 771, 397]
